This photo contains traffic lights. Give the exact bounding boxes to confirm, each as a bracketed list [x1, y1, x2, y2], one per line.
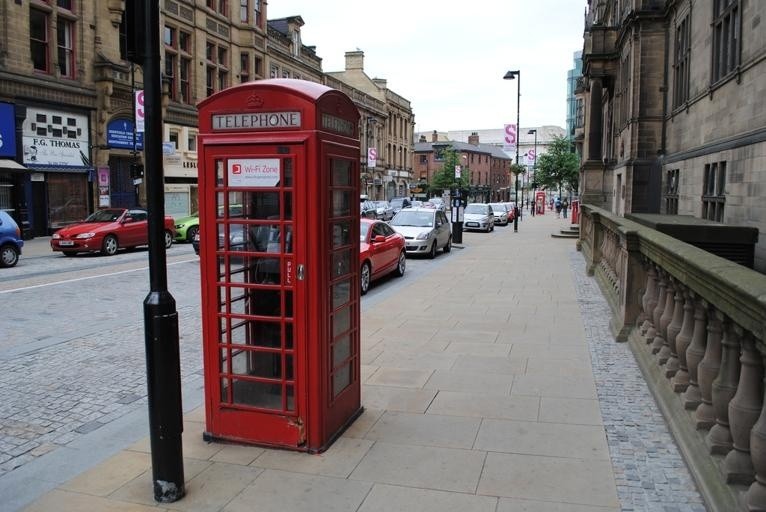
[136, 164, 144, 180]
[130, 164, 138, 180]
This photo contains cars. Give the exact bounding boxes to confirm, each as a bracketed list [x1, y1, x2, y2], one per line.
[503, 200, 529, 223]
[0, 209, 24, 268]
[387, 206, 453, 259]
[459, 203, 495, 233]
[193, 218, 249, 262]
[50, 207, 175, 258]
[359, 194, 446, 222]
[172, 204, 247, 243]
[359, 217, 408, 296]
[487, 203, 508, 227]
[49, 196, 86, 217]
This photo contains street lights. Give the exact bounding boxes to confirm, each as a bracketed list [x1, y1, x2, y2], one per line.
[423, 159, 429, 203]
[452, 150, 467, 207]
[528, 130, 537, 215]
[504, 70, 520, 232]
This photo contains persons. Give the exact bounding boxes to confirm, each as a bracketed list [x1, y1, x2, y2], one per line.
[555, 198, 561, 218]
[549, 198, 554, 211]
[528, 198, 536, 217]
[539, 199, 541, 211]
[561, 198, 569, 218]
